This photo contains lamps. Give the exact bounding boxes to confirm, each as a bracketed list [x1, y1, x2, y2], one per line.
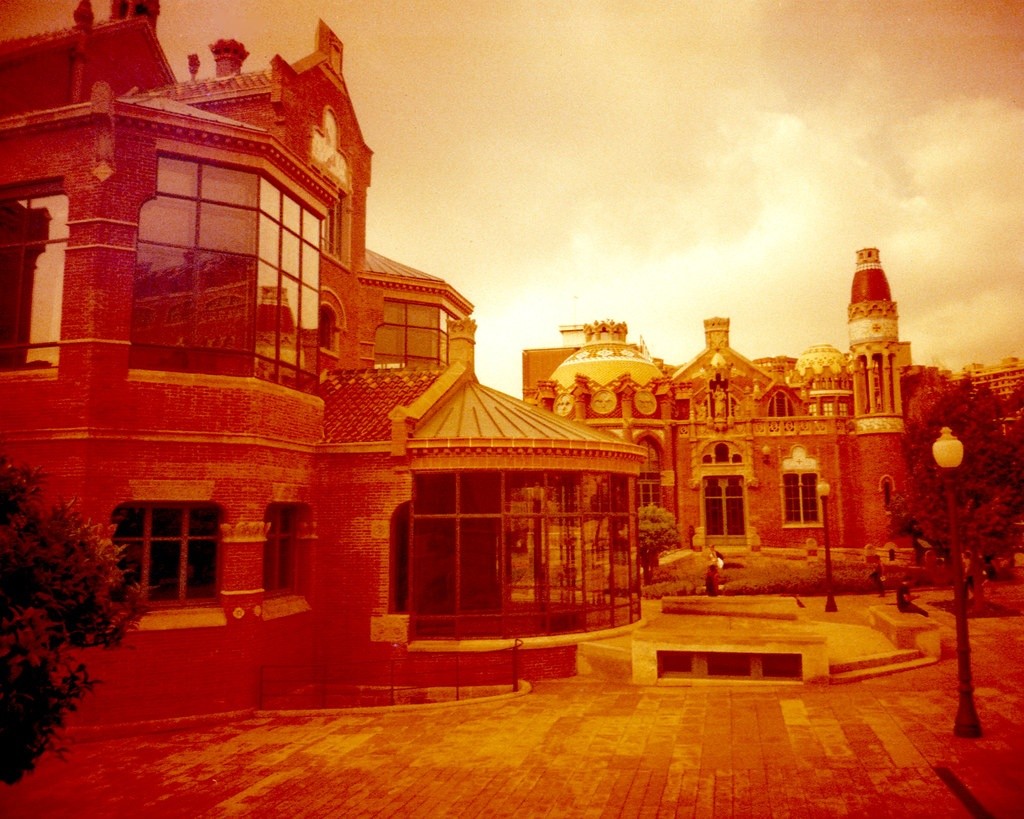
[761, 443, 772, 461]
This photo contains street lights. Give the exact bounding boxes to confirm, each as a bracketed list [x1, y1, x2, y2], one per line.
[931, 424, 984, 739]
[814, 479, 840, 612]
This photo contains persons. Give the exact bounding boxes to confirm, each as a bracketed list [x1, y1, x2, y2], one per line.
[896, 574, 929, 617]
[705, 544, 719, 597]
[982, 549, 997, 580]
[963, 551, 976, 600]
[869, 554, 887, 597]
